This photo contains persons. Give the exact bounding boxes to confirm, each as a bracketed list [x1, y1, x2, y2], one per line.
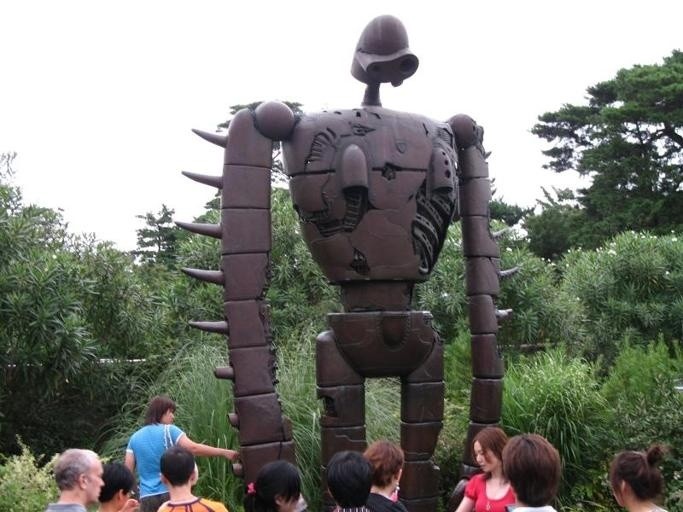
[324, 450, 377, 512]
[501, 434, 560, 512]
[454, 427, 515, 512]
[607, 443, 670, 512]
[243, 460, 302, 512]
[363, 440, 408, 511]
[124, 395, 240, 512]
[43, 447, 106, 512]
[96, 463, 139, 511]
[157, 445, 227, 512]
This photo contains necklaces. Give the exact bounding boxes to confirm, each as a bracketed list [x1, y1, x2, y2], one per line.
[486, 486, 501, 511]
